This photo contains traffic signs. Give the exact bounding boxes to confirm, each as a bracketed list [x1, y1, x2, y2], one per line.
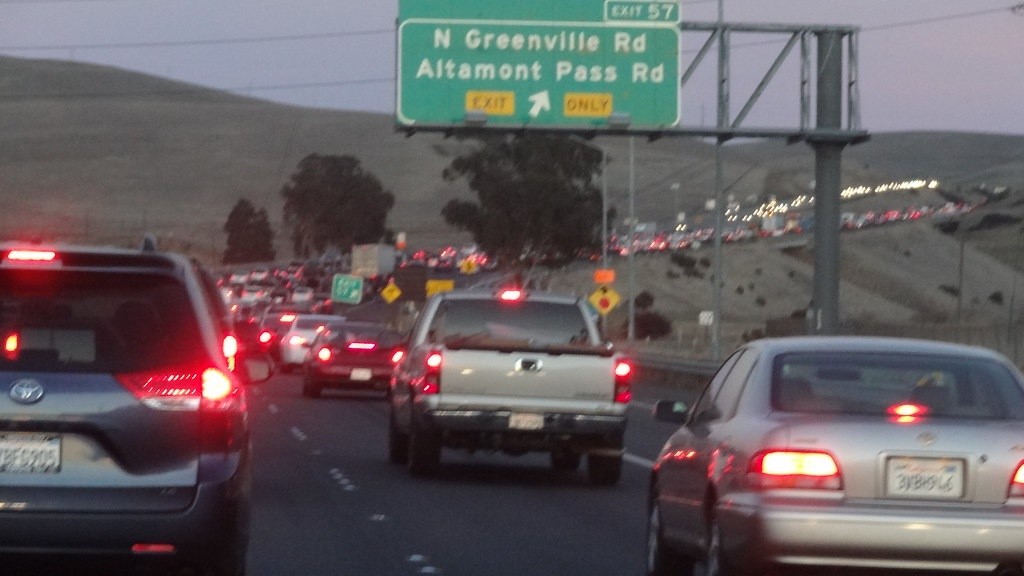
[395, 1, 682, 129]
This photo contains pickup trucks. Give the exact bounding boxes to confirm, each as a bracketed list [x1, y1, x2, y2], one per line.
[386, 293, 633, 488]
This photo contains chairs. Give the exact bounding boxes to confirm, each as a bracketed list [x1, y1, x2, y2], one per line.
[99, 300, 166, 370]
[909, 370, 954, 407]
[780, 379, 849, 415]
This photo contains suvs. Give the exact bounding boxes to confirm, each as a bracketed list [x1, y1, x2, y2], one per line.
[1, 232, 275, 576]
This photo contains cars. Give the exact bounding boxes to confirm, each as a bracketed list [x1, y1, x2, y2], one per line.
[303, 322, 405, 398]
[644, 336, 1023, 576]
[211, 198, 977, 372]
